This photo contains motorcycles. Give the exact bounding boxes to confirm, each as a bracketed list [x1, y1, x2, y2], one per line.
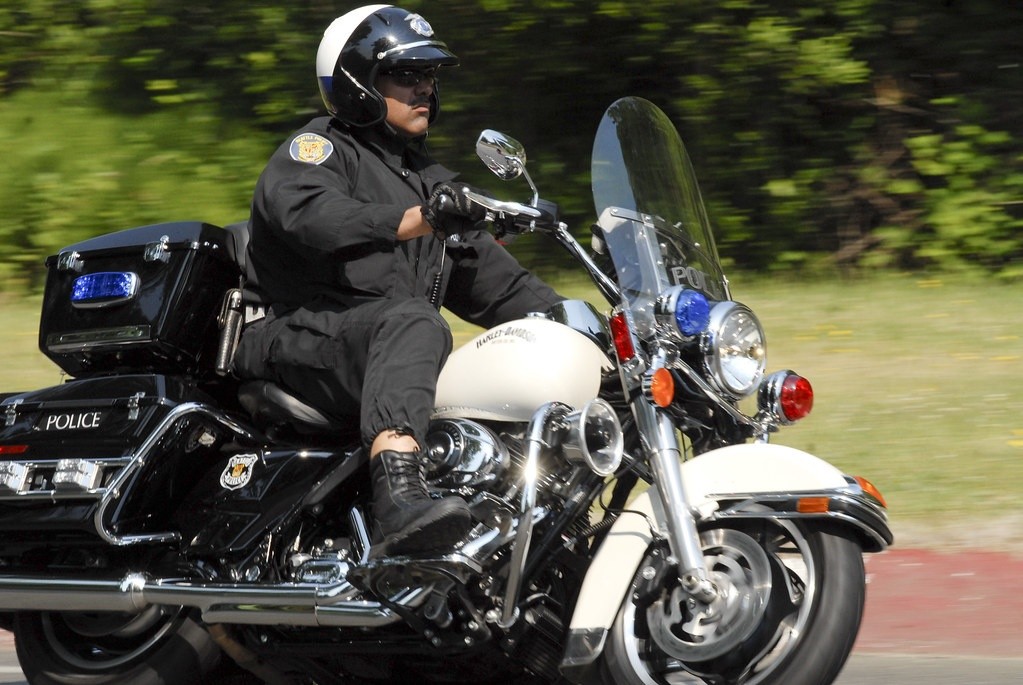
[0, 88, 895, 685]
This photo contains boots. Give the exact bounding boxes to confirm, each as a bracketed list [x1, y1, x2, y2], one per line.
[367, 445, 471, 562]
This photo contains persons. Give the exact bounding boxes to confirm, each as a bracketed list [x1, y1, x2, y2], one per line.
[247, 3, 571, 561]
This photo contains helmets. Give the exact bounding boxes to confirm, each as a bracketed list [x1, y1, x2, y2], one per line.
[315, 4, 460, 128]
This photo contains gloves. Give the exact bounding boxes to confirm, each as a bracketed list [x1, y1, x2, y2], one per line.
[420, 182, 507, 242]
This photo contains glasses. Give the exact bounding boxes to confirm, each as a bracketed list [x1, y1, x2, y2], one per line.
[376, 65, 439, 87]
